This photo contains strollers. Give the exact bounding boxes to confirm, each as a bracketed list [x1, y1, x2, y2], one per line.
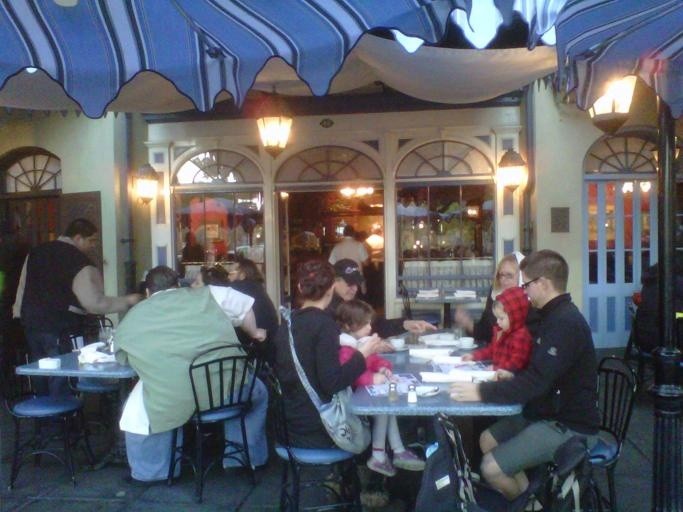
[414, 411, 593, 511]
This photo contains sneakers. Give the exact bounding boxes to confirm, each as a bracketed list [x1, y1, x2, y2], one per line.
[334, 259, 366, 286]
[365, 455, 397, 477]
[392, 449, 425, 472]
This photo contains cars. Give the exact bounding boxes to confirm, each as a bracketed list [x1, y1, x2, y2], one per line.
[172, 244, 262, 263]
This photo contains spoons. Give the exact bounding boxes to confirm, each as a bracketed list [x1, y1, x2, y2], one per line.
[422, 385, 440, 395]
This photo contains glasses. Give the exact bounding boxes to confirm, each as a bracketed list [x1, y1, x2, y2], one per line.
[521, 277, 540, 290]
[497, 272, 515, 280]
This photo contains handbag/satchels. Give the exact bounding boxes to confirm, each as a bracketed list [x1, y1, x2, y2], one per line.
[319, 386, 372, 455]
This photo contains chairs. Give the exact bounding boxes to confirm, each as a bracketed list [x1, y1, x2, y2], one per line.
[254, 358, 364, 511]
[54, 310, 118, 463]
[622, 300, 654, 407]
[578, 356, 641, 512]
[165, 343, 257, 503]
[0, 340, 89, 490]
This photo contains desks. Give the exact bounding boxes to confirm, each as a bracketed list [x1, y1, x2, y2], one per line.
[345, 324, 524, 444]
[16, 345, 136, 466]
[395, 286, 489, 331]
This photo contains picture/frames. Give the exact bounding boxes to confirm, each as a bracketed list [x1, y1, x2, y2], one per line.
[200, 219, 224, 243]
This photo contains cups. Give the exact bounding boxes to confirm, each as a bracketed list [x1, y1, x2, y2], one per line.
[97, 326, 111, 345]
[459, 336, 474, 346]
[389, 338, 404, 348]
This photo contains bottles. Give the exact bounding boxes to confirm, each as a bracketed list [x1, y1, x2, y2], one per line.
[407, 384, 417, 404]
[388, 384, 399, 401]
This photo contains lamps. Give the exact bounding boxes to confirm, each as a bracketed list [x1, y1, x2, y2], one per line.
[498, 140, 527, 198]
[250, 85, 292, 160]
[128, 159, 160, 210]
[589, 73, 635, 137]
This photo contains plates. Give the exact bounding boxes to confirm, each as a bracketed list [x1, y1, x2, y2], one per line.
[391, 346, 409, 351]
[416, 385, 440, 397]
[456, 343, 478, 350]
